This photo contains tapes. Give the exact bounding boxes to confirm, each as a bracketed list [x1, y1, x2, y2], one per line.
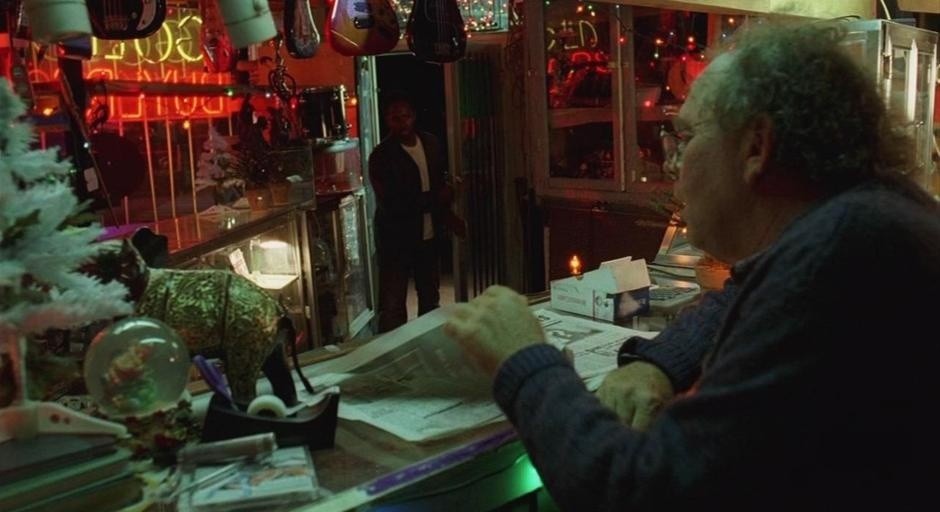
[247, 385, 341, 418]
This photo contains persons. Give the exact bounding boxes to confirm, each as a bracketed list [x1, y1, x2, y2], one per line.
[367, 96, 455, 331]
[445, 24, 939, 510]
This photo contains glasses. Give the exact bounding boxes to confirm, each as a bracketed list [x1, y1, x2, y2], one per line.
[660, 117, 715, 166]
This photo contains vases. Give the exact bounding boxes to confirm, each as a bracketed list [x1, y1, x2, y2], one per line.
[217, 191, 236, 228]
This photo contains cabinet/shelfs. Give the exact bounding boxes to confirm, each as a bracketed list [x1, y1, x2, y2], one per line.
[245, 180, 380, 340]
[512, 0, 738, 289]
[109, 232, 694, 511]
[116, 205, 308, 378]
[832, 21, 939, 197]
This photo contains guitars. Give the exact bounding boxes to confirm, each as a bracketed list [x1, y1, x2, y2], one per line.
[85, 0, 166, 39]
[405, 1, 467, 62]
[327, 0, 400, 56]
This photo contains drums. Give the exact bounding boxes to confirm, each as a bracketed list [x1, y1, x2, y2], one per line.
[313, 137, 361, 197]
[296, 83, 352, 141]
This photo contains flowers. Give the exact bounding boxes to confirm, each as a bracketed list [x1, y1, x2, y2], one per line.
[194, 124, 267, 195]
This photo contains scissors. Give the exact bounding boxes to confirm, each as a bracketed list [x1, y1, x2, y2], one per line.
[193, 354, 240, 412]
